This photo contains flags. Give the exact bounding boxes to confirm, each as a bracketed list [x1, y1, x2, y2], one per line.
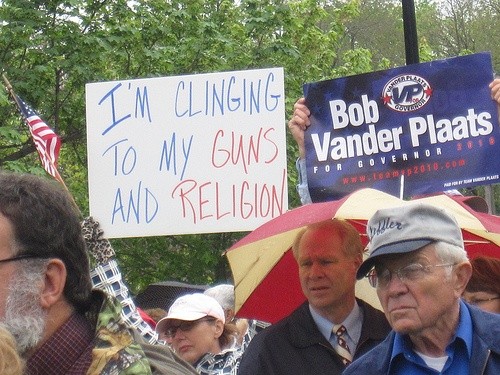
[7, 86, 61, 181]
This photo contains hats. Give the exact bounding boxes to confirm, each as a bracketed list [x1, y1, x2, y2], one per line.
[354, 202, 465, 281]
[155, 291, 227, 336]
[410, 189, 489, 214]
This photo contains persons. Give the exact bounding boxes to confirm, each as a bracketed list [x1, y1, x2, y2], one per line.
[203, 285, 236, 325]
[289, 78, 500, 205]
[80, 216, 271, 375]
[0, 324, 23, 375]
[238, 220, 392, 375]
[144, 308, 168, 324]
[342, 201, 500, 375]
[462, 258, 500, 314]
[0, 170, 200, 374]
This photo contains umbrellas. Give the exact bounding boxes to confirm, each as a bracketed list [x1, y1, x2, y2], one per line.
[221, 187, 500, 334]
[134, 280, 207, 312]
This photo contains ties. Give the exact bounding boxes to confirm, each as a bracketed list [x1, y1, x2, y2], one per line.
[332, 324, 353, 367]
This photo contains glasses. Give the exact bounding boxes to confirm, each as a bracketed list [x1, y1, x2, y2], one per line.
[364, 260, 457, 290]
[461, 293, 500, 307]
[165, 317, 214, 337]
